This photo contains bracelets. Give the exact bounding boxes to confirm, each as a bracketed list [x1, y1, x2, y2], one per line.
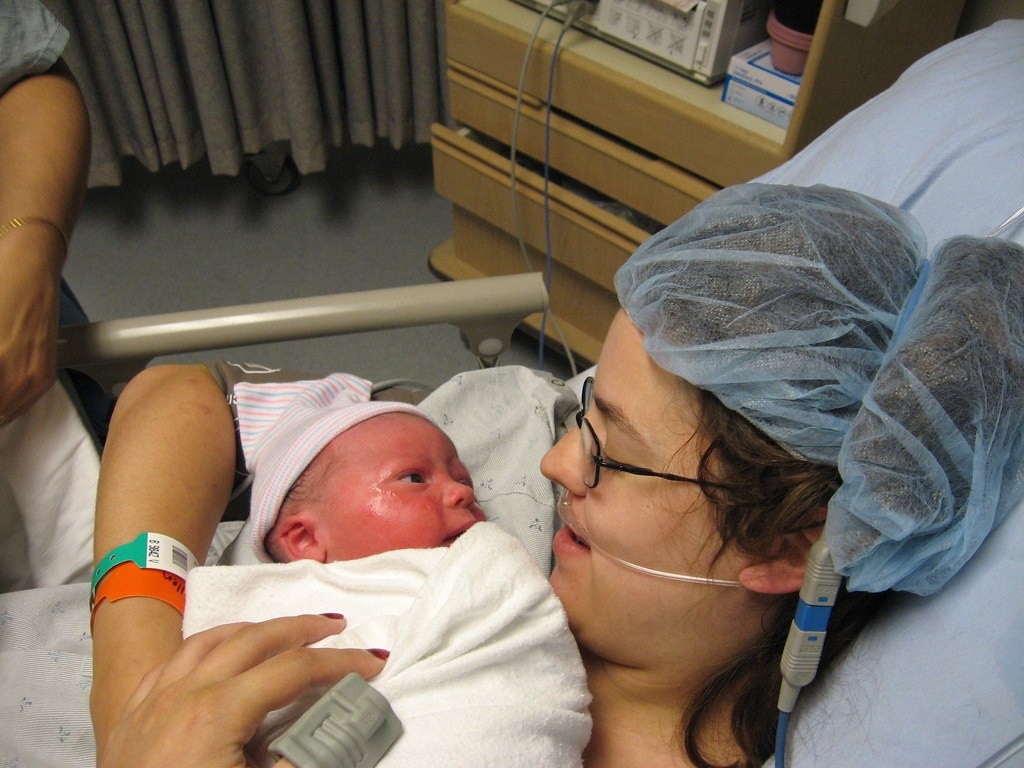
[0, 217, 70, 260]
[90, 532, 199, 639]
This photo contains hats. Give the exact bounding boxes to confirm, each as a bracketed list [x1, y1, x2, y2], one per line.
[230, 373, 440, 563]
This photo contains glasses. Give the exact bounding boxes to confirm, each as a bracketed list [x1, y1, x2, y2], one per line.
[575, 377, 739, 492]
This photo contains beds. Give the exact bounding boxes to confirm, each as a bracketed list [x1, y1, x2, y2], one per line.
[0, 269, 600, 768]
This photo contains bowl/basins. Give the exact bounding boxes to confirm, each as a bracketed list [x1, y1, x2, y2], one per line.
[767, 7, 814, 75]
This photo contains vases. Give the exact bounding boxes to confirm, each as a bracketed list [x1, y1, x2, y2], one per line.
[766, 7, 814, 75]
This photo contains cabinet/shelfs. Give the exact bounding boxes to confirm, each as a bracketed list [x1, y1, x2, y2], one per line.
[426, 0, 968, 366]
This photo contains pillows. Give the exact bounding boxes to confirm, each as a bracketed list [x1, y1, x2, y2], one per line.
[749, 18, 1024, 767]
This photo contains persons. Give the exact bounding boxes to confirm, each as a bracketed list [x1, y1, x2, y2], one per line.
[89, 182, 1024, 768]
[0, 0, 124, 594]
[182, 399, 595, 768]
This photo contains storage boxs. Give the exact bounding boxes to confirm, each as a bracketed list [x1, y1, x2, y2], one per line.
[722, 39, 803, 130]
